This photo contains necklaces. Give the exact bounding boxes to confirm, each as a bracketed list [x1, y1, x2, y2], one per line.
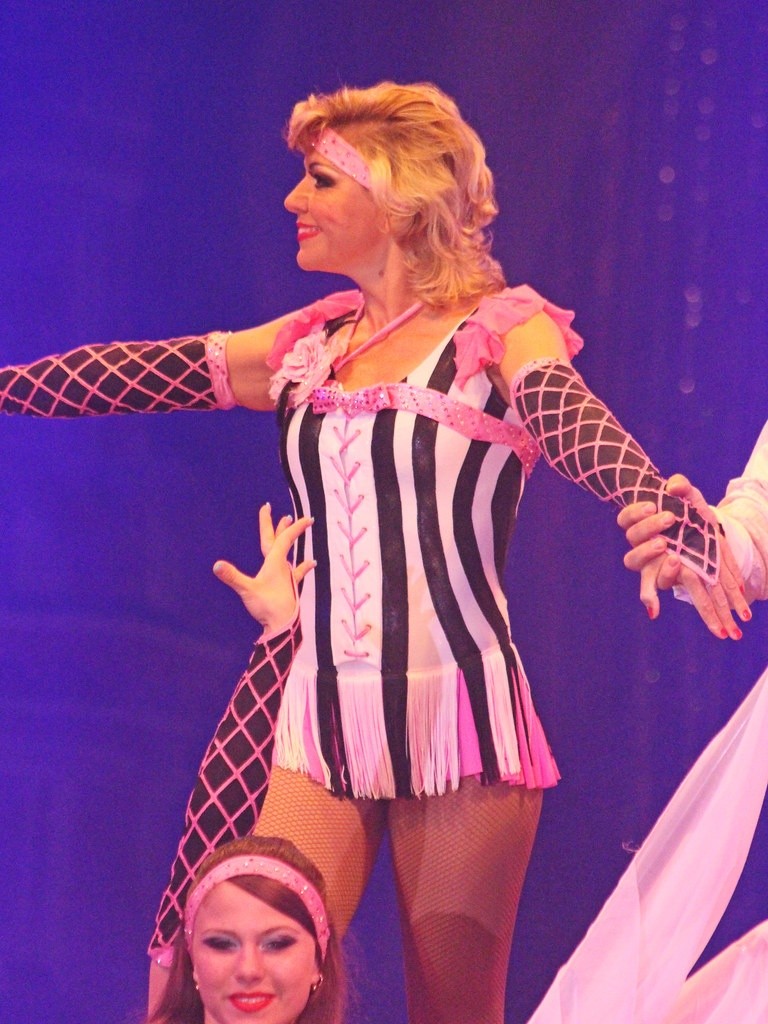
[333, 297, 426, 374]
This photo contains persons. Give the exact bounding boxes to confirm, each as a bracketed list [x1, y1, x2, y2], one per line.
[617, 420, 768, 603]
[0, 81, 752, 1024]
